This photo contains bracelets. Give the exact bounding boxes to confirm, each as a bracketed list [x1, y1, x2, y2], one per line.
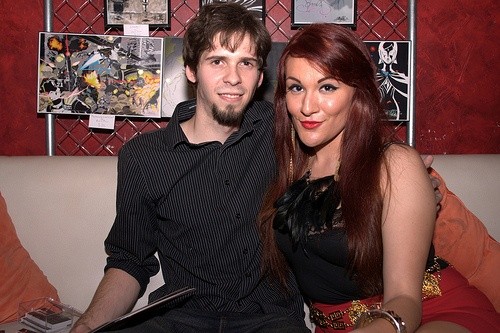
[350, 308, 408, 333]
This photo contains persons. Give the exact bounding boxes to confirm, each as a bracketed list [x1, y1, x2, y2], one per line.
[256, 23, 500, 333]
[69, 3, 444, 333]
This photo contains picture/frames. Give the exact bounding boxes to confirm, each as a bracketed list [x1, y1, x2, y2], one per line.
[199, 0, 267, 31]
[104, 0, 171, 30]
[291, 0, 357, 30]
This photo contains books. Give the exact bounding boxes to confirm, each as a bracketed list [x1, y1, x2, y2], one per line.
[0, 285, 197, 333]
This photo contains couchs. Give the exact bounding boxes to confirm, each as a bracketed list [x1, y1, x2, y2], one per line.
[0, 156, 500, 333]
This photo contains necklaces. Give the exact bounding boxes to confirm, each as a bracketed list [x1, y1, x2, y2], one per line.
[301, 154, 340, 201]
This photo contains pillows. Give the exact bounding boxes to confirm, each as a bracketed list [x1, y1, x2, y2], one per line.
[0, 193, 60, 324]
[430, 166, 500, 314]
